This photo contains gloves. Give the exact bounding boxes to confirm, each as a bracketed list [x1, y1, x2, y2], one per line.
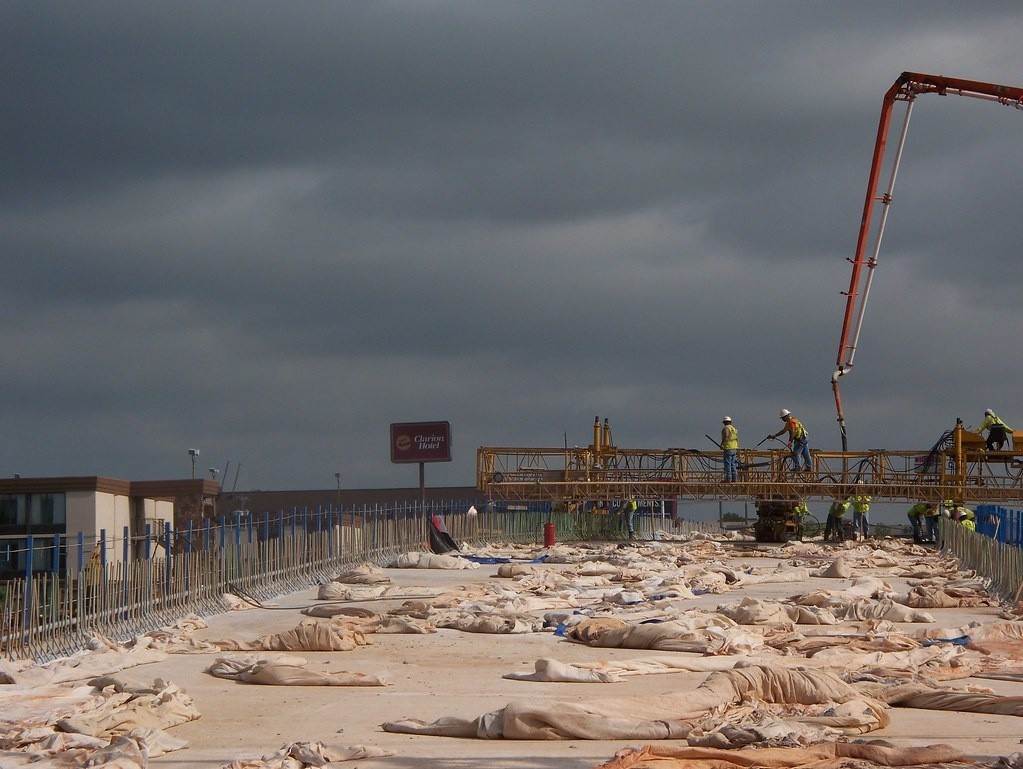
[616, 511, 621, 517]
[720, 443, 724, 450]
[806, 511, 812, 516]
[767, 434, 776, 440]
[788, 441, 791, 448]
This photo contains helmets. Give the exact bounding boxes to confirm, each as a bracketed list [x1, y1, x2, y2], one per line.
[779, 409, 791, 419]
[956, 511, 967, 519]
[985, 409, 994, 414]
[723, 416, 731, 421]
[943, 510, 950, 517]
[858, 480, 865, 487]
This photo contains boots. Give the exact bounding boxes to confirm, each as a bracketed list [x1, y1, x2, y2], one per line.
[840, 533, 845, 542]
[824, 533, 829, 542]
[628, 532, 636, 541]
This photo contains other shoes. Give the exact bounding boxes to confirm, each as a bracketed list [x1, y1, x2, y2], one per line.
[929, 539, 932, 543]
[804, 469, 811, 473]
[720, 479, 732, 483]
[791, 467, 801, 473]
[732, 480, 737, 482]
[935, 540, 938, 543]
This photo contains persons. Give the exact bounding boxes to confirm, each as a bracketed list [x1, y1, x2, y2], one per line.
[768, 408, 813, 472]
[850, 480, 872, 540]
[616, 493, 637, 539]
[975, 408, 1016, 450]
[824, 495, 852, 543]
[720, 416, 739, 483]
[907, 499, 978, 545]
[793, 495, 809, 523]
